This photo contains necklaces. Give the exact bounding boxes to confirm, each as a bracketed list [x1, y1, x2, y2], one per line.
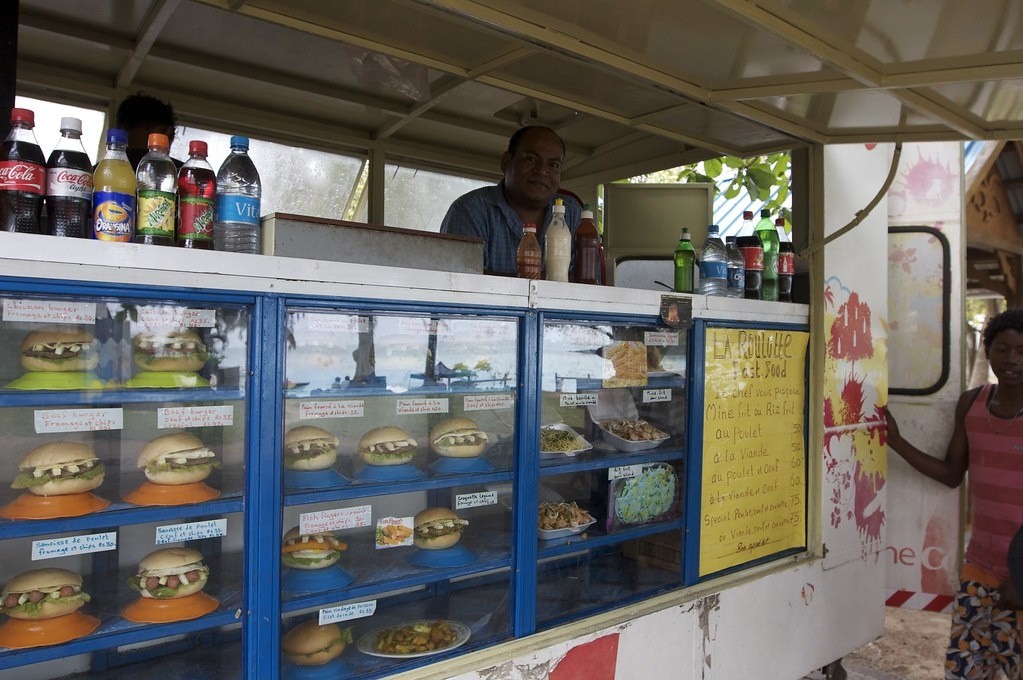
[987, 386, 1023, 433]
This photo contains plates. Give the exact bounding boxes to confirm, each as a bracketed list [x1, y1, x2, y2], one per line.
[615, 462, 682, 526]
[356, 618, 472, 659]
[599, 421, 671, 452]
[537, 424, 593, 459]
[535, 514, 597, 540]
[646, 368, 678, 381]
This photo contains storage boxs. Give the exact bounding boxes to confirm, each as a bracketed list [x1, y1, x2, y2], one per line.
[260, 211, 487, 275]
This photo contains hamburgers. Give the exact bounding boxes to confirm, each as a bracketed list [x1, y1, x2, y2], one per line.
[281, 620, 356, 666]
[359, 426, 417, 464]
[284, 425, 339, 471]
[1, 567, 90, 619]
[414, 506, 469, 549]
[137, 432, 222, 484]
[284, 526, 346, 570]
[22, 327, 100, 372]
[128, 548, 210, 598]
[431, 418, 489, 457]
[10, 442, 106, 496]
[133, 325, 207, 372]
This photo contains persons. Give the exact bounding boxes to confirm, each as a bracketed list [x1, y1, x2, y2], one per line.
[440, 126, 602, 285]
[883, 309, 1023, 680]
[87, 90, 185, 241]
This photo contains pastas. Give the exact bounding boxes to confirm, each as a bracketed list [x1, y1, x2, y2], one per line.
[539, 424, 586, 453]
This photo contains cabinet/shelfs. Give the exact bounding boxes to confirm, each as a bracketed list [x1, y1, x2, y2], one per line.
[0, 260, 703, 680]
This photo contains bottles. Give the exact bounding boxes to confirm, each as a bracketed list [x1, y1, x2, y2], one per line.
[573, 202, 600, 285]
[0, 107, 47, 237]
[214, 135, 261, 256]
[543, 197, 572, 284]
[136, 133, 178, 248]
[176, 139, 216, 251]
[675, 208, 795, 303]
[47, 117, 94, 240]
[91, 127, 137, 245]
[516, 223, 541, 281]
